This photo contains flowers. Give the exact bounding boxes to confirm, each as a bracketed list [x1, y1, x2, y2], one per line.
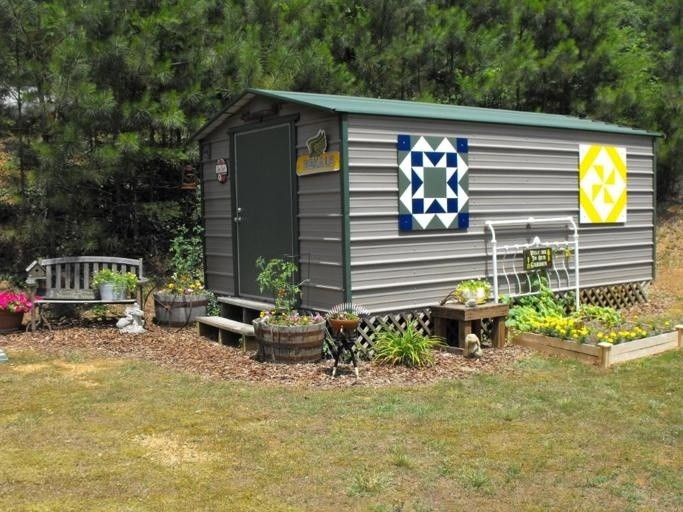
[89, 265, 210, 297]
[0, 288, 41, 315]
[250, 251, 323, 328]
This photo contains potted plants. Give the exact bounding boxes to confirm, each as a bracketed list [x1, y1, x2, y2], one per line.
[331, 312, 360, 338]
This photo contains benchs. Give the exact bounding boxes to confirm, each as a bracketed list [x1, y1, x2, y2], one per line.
[34, 253, 143, 332]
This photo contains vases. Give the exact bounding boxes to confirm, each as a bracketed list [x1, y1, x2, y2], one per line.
[98, 282, 213, 327]
[250, 317, 325, 364]
[0, 310, 24, 334]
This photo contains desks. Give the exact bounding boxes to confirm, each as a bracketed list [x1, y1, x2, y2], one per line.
[433, 303, 510, 350]
